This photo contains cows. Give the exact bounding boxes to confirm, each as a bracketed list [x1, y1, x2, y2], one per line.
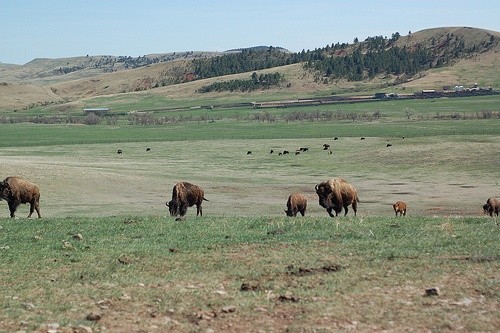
[296, 152, 300, 155]
[164, 182, 209, 217]
[387, 144, 391, 147]
[146, 148, 150, 151]
[279, 152, 281, 155]
[283, 151, 289, 154]
[361, 137, 365, 140]
[300, 148, 304, 151]
[315, 179, 361, 218]
[303, 148, 308, 151]
[247, 151, 251, 154]
[117, 150, 122, 152]
[393, 201, 407, 214]
[0, 177, 41, 219]
[283, 193, 308, 217]
[270, 150, 273, 153]
[334, 136, 338, 140]
[483, 197, 500, 216]
[323, 144, 333, 154]
